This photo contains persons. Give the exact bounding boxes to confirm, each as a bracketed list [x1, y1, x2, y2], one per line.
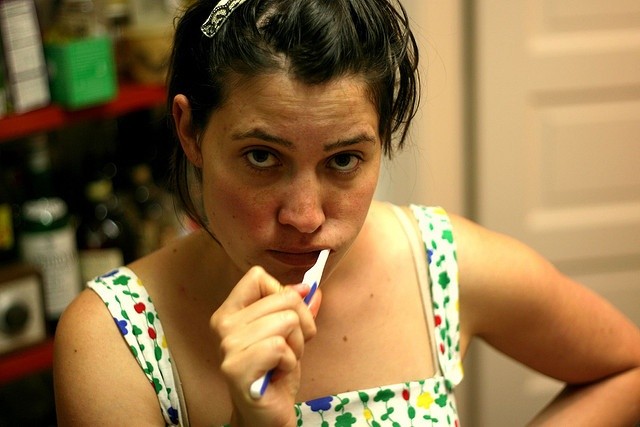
[51, 0, 640, 427]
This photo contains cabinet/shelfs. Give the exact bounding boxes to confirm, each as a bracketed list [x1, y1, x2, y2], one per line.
[0, 73, 169, 384]
